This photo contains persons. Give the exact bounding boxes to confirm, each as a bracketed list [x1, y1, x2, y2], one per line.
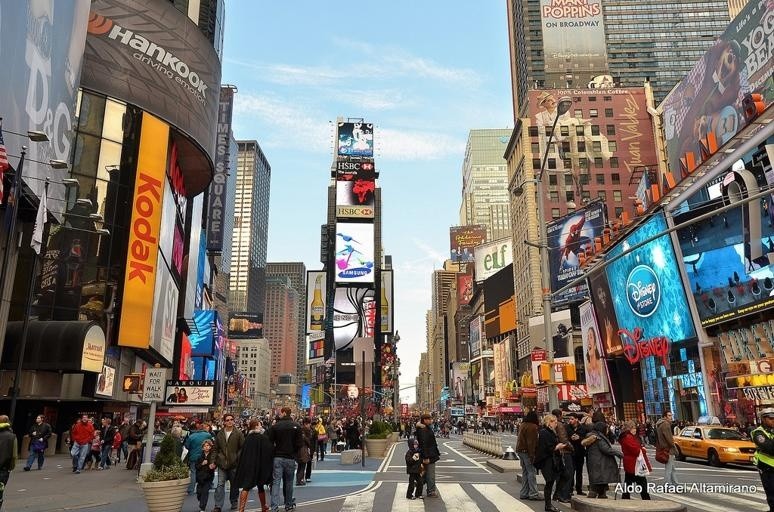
[517, 407, 677, 510]
[195, 439, 215, 512]
[266, 407, 302, 512]
[208, 413, 245, 512]
[534, 91, 570, 122]
[0, 414, 17, 512]
[414, 413, 440, 497]
[451, 245, 474, 262]
[678, 415, 763, 447]
[405, 437, 425, 500]
[749, 407, 773, 512]
[562, 214, 592, 261]
[64, 380, 406, 494]
[595, 283, 619, 351]
[22, 414, 52, 470]
[408, 403, 523, 441]
[455, 376, 465, 399]
[232, 418, 272, 512]
[584, 327, 602, 388]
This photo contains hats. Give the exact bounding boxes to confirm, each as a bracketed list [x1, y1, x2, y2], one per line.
[421, 414, 433, 419]
[757, 408, 774, 418]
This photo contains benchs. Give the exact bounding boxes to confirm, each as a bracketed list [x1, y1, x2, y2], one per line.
[341, 449, 362, 464]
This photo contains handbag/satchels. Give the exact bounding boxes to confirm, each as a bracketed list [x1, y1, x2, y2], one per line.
[634, 450, 650, 477]
[32, 439, 46, 452]
[655, 446, 669, 464]
[552, 452, 574, 476]
[318, 434, 327, 441]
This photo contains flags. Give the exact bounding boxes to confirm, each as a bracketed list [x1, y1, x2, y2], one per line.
[0, 121, 8, 207]
[28, 183, 48, 257]
[3, 152, 25, 240]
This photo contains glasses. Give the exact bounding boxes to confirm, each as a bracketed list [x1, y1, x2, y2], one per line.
[226, 419, 234, 422]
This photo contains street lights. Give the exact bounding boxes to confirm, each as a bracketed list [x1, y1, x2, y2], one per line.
[511, 96, 573, 416]
[294, 399, 309, 411]
[295, 385, 334, 416]
[422, 370, 434, 411]
[391, 330, 400, 427]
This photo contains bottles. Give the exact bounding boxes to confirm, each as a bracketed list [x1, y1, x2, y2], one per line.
[228, 319, 263, 331]
[64, 237, 83, 289]
[310, 280, 323, 330]
[379, 274, 388, 331]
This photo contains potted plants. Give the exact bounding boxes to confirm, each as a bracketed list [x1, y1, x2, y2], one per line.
[132, 461, 194, 512]
[391, 420, 399, 444]
[363, 418, 389, 460]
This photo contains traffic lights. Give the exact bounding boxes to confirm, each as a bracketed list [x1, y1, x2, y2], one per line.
[123, 375, 142, 393]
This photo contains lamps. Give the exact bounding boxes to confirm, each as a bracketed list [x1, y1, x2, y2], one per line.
[0, 126, 110, 238]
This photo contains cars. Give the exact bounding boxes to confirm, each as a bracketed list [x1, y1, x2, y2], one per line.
[673, 425, 756, 468]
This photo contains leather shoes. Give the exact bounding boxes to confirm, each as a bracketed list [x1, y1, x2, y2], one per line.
[545, 505, 561, 512]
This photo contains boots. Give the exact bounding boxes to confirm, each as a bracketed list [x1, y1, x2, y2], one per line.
[259, 491, 269, 512]
